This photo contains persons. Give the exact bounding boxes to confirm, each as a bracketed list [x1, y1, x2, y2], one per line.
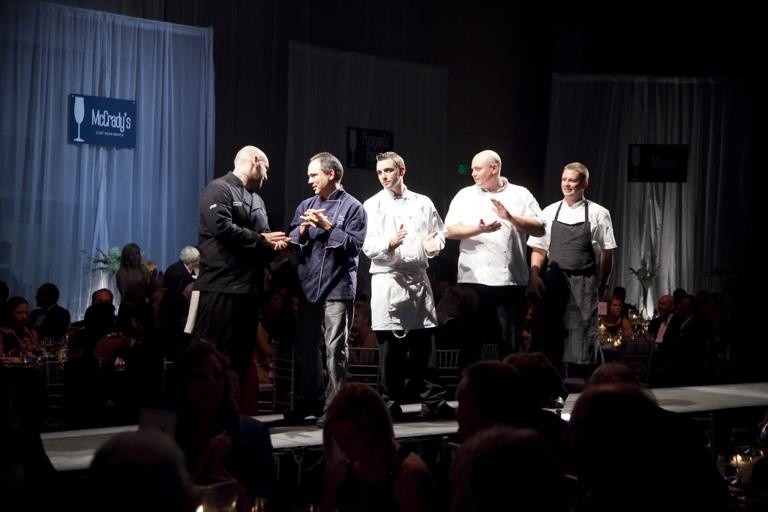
[198, 145, 290, 400]
[1, 244, 732, 512]
[362, 150, 456, 421]
[444, 150, 547, 376]
[288, 152, 367, 427]
[526, 162, 617, 384]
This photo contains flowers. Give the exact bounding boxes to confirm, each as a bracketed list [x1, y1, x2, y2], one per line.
[628, 260, 656, 307]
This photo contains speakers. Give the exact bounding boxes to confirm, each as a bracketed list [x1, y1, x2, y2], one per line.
[627, 143, 692, 184]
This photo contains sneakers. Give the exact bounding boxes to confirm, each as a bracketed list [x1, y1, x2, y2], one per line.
[390, 404, 409, 422]
[316, 414, 325, 428]
[551, 396, 565, 408]
[422, 404, 457, 421]
